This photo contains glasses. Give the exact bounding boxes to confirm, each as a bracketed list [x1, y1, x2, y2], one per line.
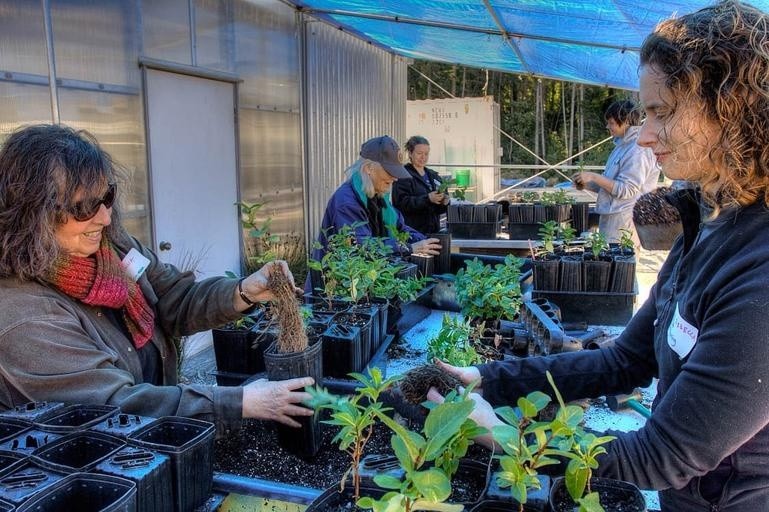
[58, 181, 119, 222]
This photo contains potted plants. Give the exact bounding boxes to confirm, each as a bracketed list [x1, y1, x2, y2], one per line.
[213, 190, 646, 512]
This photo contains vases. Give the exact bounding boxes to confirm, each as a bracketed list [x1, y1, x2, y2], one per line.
[0, 400, 217, 512]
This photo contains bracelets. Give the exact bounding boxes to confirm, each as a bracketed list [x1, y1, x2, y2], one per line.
[239, 279, 254, 306]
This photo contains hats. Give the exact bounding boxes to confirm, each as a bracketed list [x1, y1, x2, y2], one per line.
[358, 135, 414, 179]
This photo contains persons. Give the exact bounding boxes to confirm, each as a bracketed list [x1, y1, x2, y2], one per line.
[389, 133, 451, 243]
[420, 0, 769, 509]
[304, 133, 443, 298]
[570, 96, 664, 250]
[0, 123, 318, 434]
